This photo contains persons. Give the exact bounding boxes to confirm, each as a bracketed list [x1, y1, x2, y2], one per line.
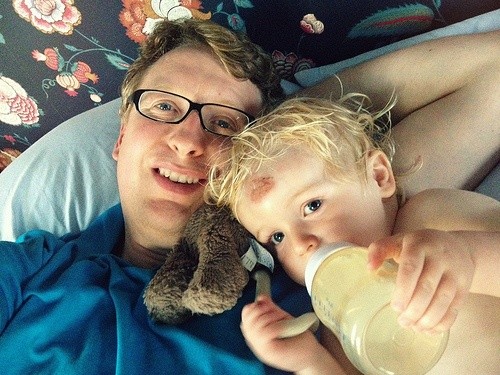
[0, 17, 500, 375]
[202, 76, 500, 375]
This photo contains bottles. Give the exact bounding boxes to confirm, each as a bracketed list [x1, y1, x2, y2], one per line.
[304, 241, 450, 375]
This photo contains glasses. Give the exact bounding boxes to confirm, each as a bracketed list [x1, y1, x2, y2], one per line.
[124, 88, 259, 138]
